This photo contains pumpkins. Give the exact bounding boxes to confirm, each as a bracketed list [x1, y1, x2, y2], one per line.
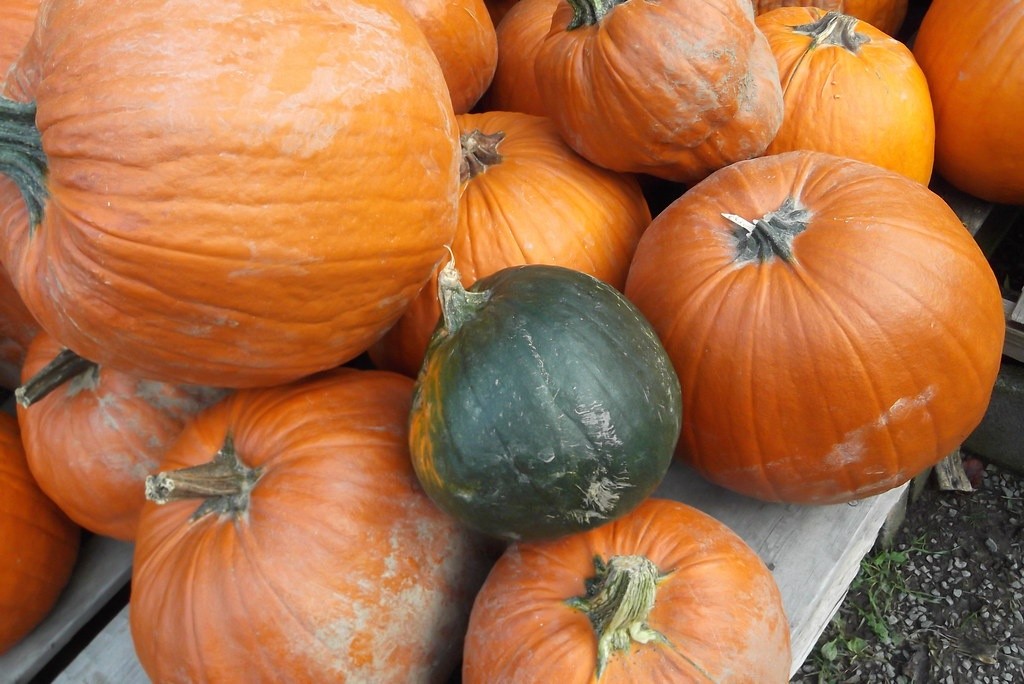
[0, 0, 1024, 684]
